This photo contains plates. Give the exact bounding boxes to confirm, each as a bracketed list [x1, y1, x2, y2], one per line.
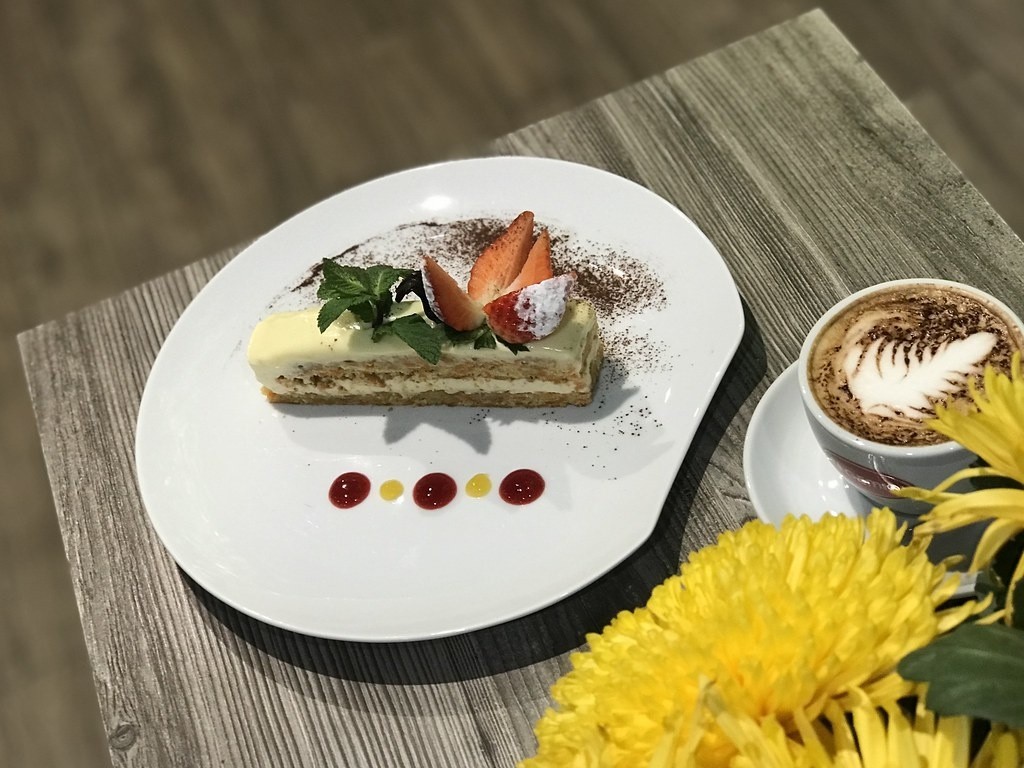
[135, 155, 746, 643]
[742, 357, 994, 599]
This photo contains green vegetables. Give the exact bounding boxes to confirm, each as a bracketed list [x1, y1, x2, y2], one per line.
[317, 257, 442, 366]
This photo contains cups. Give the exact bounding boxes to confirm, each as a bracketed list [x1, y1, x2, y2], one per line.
[798, 278, 1024, 516]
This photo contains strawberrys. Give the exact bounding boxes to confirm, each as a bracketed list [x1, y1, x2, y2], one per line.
[424, 213, 563, 345]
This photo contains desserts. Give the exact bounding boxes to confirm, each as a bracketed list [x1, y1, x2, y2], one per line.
[248, 300, 603, 409]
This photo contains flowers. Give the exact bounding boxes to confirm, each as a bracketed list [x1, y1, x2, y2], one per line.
[518, 345, 1024, 768]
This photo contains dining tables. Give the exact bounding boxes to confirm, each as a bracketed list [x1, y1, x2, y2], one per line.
[17, 7, 1024, 768]
